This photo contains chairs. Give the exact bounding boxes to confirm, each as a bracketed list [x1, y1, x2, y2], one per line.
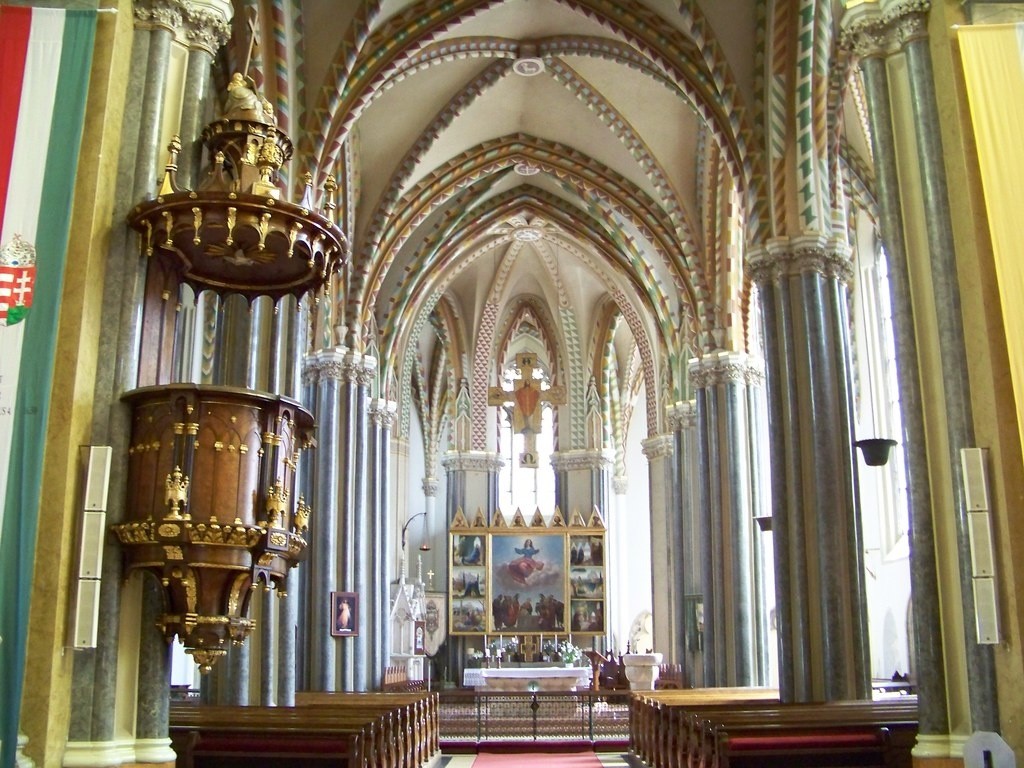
[654, 664, 683, 690]
[384, 666, 427, 694]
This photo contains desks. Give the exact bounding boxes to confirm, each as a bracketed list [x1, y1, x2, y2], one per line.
[463, 668, 594, 712]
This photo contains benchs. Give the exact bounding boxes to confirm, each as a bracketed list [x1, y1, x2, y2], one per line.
[169, 693, 441, 768]
[630, 690, 919, 768]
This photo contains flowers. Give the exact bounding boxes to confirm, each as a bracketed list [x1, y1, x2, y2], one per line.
[558, 642, 583, 664]
[467, 647, 483, 661]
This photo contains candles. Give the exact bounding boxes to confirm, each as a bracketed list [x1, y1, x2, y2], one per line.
[499, 634, 502, 649]
[484, 634, 487, 653]
[555, 634, 557, 652]
[486, 649, 490, 656]
[539, 633, 543, 653]
[497, 649, 501, 657]
[568, 633, 572, 644]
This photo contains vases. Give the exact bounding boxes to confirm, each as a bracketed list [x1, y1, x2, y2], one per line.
[468, 658, 483, 667]
[565, 663, 573, 667]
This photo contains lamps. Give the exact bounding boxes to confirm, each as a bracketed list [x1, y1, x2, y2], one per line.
[755, 355, 770, 534]
[824, 0, 898, 466]
[400, 509, 430, 550]
[330, 591, 359, 636]
